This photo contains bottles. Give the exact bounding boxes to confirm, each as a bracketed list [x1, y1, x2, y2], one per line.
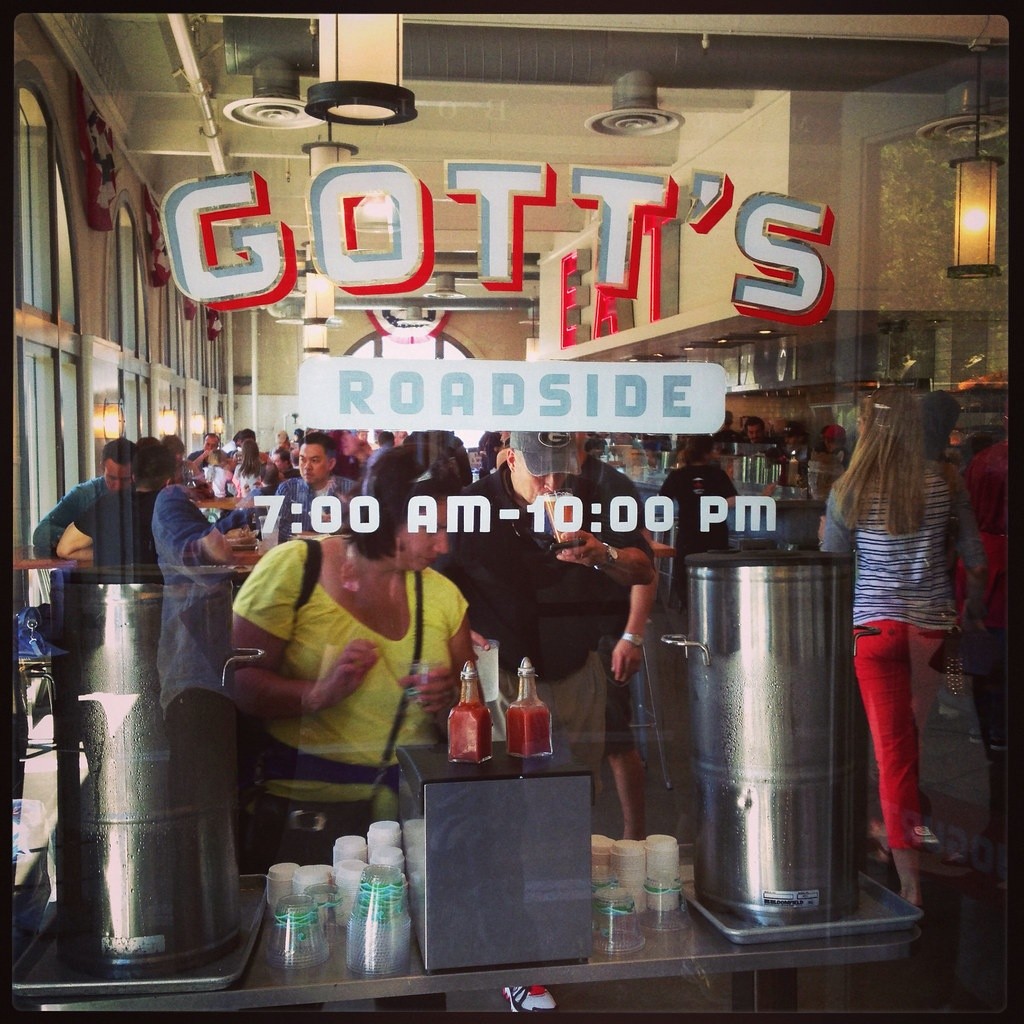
[447, 660, 493, 763]
[504, 656, 553, 757]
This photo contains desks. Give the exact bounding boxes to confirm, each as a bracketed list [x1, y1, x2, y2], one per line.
[11, 896, 930, 1012]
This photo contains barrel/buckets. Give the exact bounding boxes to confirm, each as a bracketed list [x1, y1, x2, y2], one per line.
[661, 553, 880, 922]
[32, 567, 264, 967]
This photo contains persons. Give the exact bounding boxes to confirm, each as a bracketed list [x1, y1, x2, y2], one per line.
[33, 381, 1009, 920]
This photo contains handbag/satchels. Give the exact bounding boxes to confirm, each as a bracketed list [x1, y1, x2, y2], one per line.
[17, 606, 70, 659]
[244, 790, 378, 874]
[939, 640, 977, 699]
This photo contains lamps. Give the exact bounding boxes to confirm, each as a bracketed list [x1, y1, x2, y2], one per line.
[219, 15, 674, 353]
[946, 39, 1002, 281]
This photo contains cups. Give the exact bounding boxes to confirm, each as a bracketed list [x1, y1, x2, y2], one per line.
[266, 821, 412, 978]
[590, 834, 690, 955]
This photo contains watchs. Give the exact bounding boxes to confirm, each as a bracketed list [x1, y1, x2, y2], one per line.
[622, 633, 644, 647]
[594, 539, 618, 571]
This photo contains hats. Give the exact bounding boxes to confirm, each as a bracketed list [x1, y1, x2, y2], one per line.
[275, 431, 287, 443]
[509, 431, 582, 477]
[825, 425, 846, 439]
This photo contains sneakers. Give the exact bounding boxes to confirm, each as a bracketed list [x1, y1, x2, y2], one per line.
[502, 986, 559, 1012]
